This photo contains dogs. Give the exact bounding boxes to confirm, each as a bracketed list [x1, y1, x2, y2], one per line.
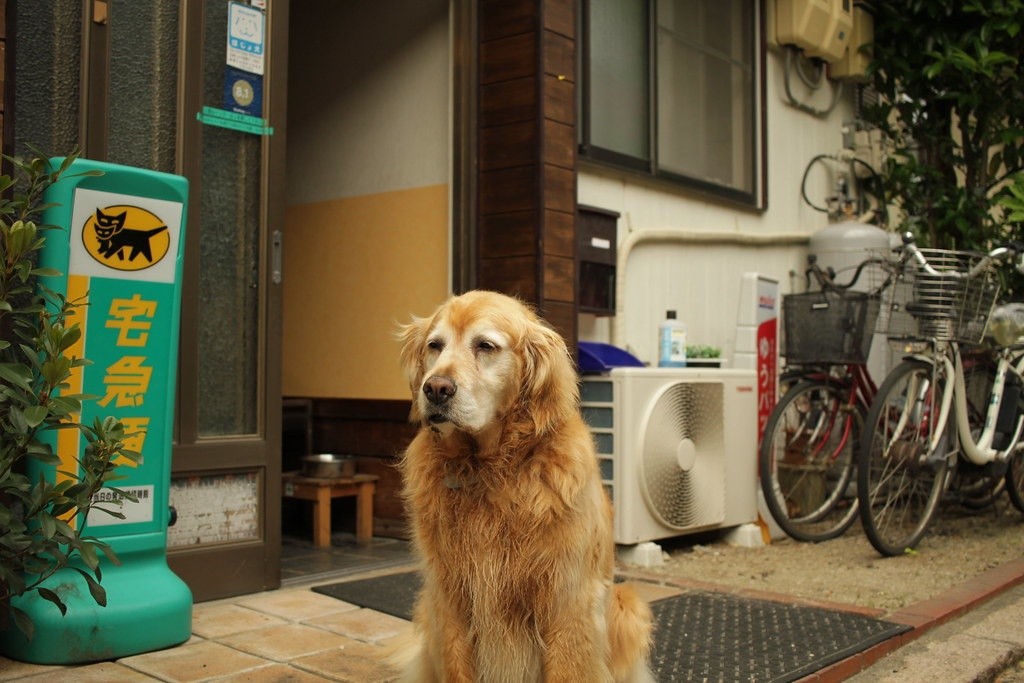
[394, 291, 663, 683]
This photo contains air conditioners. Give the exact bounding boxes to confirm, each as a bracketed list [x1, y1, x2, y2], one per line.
[580, 366, 758, 545]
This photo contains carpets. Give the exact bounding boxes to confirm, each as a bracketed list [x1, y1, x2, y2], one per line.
[311, 570, 421, 622]
[650, 587, 914, 683]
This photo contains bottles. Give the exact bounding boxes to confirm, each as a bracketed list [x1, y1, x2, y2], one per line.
[659, 311, 686, 366]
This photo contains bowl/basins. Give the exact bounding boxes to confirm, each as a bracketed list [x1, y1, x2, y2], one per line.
[303, 454, 356, 478]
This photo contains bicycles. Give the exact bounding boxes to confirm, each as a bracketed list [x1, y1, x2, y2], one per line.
[854, 230, 1024, 556]
[760, 252, 1012, 543]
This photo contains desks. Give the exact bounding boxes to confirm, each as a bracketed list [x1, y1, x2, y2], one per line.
[281, 473, 380, 549]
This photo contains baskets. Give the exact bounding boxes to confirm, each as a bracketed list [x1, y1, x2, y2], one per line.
[783, 291, 882, 366]
[886, 247, 1001, 346]
